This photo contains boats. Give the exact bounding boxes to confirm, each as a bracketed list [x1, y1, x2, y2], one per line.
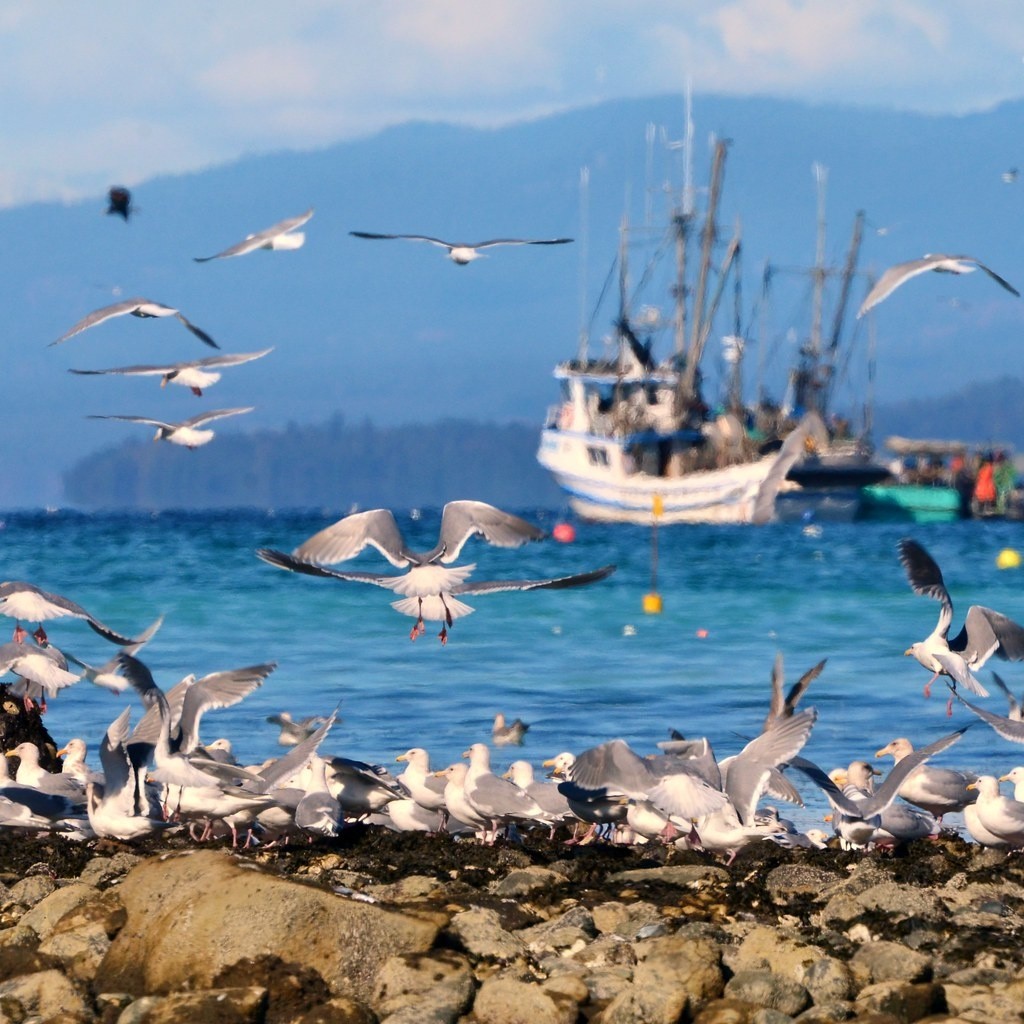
[855, 432, 970, 520]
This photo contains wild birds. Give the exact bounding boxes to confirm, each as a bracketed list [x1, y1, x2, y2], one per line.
[351, 231, 573, 264]
[855, 254, 1020, 320]
[193, 210, 314, 264]
[0, 500, 1023, 867]
[106, 186, 132, 221]
[49, 300, 218, 348]
[68, 347, 272, 396]
[87, 407, 252, 450]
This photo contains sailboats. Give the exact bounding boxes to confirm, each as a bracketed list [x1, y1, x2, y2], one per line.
[533, 68, 818, 528]
[774, 208, 891, 488]
